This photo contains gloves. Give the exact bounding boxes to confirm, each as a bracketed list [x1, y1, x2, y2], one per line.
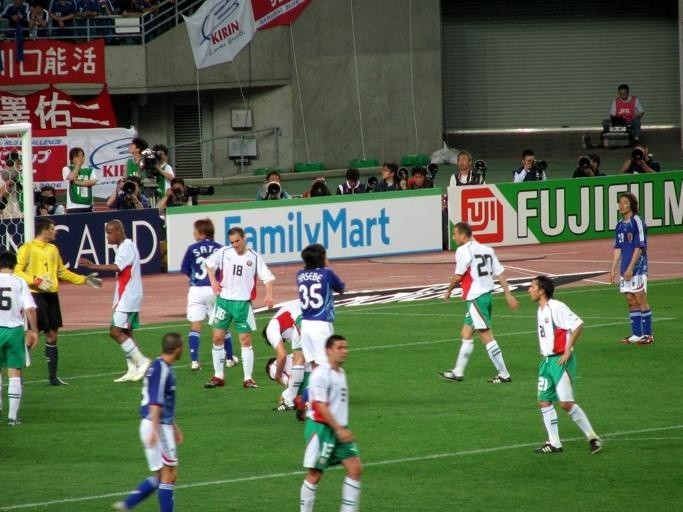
[85, 272, 103, 289]
[34, 276, 52, 291]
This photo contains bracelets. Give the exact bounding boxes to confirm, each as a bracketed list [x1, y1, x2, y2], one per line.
[447, 286, 454, 290]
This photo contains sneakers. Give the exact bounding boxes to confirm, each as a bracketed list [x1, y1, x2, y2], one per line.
[487, 374, 512, 383]
[243, 378, 258, 388]
[113, 358, 151, 382]
[111, 500, 129, 511]
[533, 441, 564, 454]
[204, 376, 224, 388]
[589, 434, 604, 456]
[620, 335, 654, 345]
[272, 395, 306, 421]
[191, 361, 200, 370]
[226, 355, 238, 368]
[438, 369, 464, 383]
[8, 416, 23, 425]
[49, 377, 68, 385]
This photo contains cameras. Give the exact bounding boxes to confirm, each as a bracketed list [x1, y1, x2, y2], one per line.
[172, 187, 182, 195]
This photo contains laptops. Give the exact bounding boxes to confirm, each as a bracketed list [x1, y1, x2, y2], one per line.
[611, 116, 630, 126]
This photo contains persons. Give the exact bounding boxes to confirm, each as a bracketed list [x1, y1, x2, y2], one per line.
[399, 165, 435, 189]
[336, 169, 367, 194]
[374, 162, 408, 190]
[293, 243, 345, 421]
[262, 299, 310, 411]
[205, 227, 275, 389]
[436, 220, 521, 386]
[180, 219, 238, 370]
[108, 331, 185, 512]
[301, 173, 331, 198]
[1, 138, 198, 273]
[78, 220, 153, 383]
[1, 251, 38, 425]
[599, 82, 645, 145]
[0, 0, 198, 47]
[618, 143, 659, 174]
[13, 218, 103, 386]
[525, 273, 604, 456]
[449, 149, 487, 185]
[608, 191, 656, 347]
[256, 171, 289, 202]
[298, 333, 365, 512]
[571, 152, 607, 177]
[511, 147, 548, 184]
